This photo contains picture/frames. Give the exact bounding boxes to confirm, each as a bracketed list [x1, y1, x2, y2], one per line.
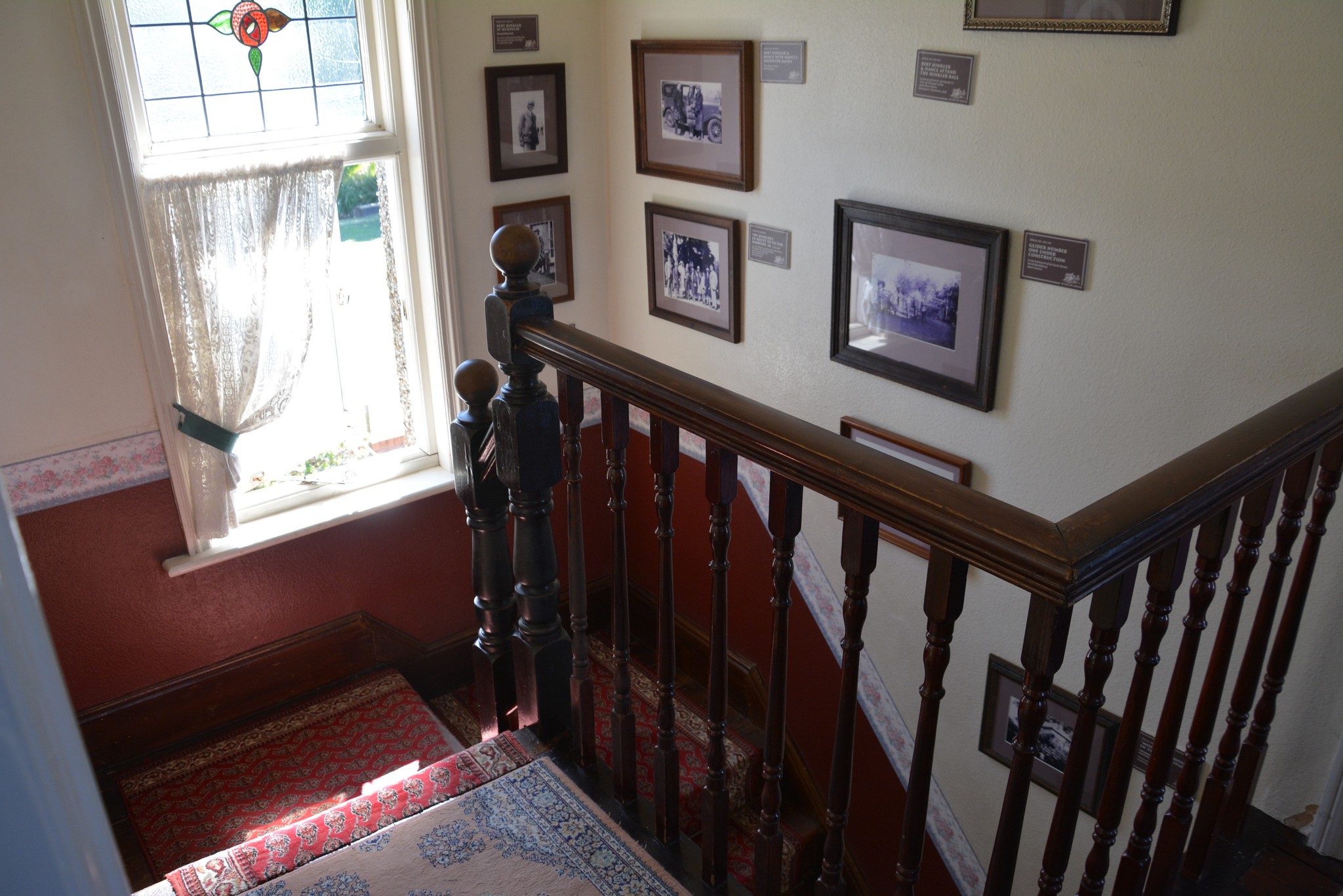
[829, 199, 1008, 412]
[644, 202, 741, 344]
[837, 416, 971, 560]
[964, 0, 1172, 33]
[630, 40, 753, 193]
[484, 63, 568, 183]
[493, 196, 575, 305]
[978, 653, 1122, 820]
